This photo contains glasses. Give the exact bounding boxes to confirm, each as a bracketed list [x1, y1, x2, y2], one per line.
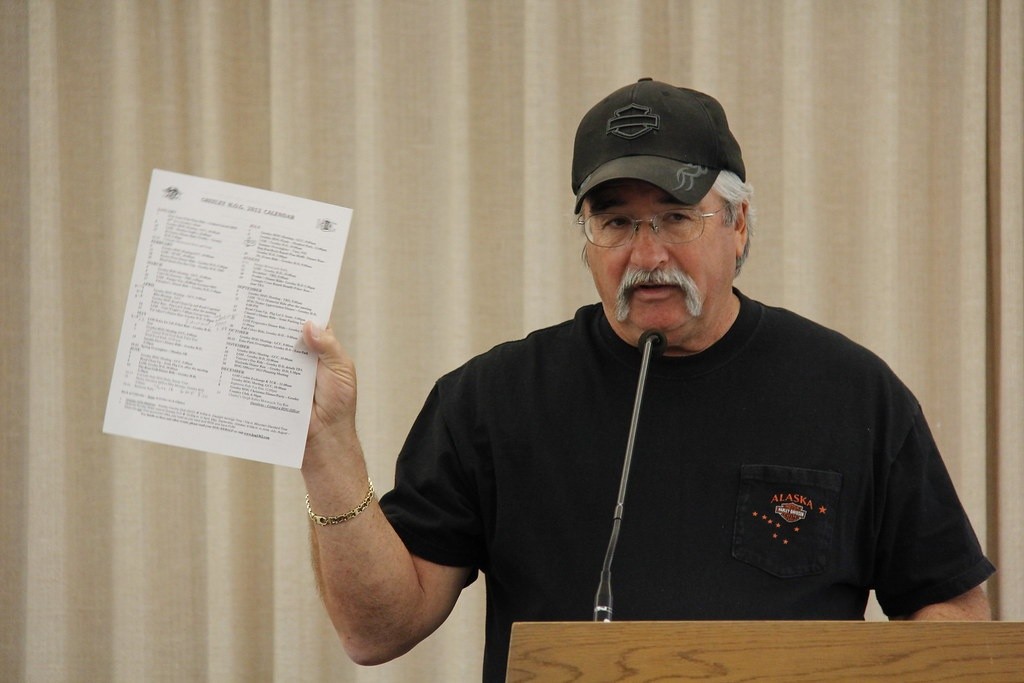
[578, 204, 729, 247]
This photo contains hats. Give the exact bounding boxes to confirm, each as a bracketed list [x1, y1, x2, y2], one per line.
[572, 77, 746, 215]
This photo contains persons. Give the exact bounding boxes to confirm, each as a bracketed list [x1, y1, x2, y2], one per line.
[300, 77, 996, 683]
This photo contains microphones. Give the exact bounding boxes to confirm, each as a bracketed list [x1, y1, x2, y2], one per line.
[594, 329, 669, 622]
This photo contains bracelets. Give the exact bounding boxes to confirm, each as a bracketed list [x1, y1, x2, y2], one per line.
[305, 477, 374, 527]
